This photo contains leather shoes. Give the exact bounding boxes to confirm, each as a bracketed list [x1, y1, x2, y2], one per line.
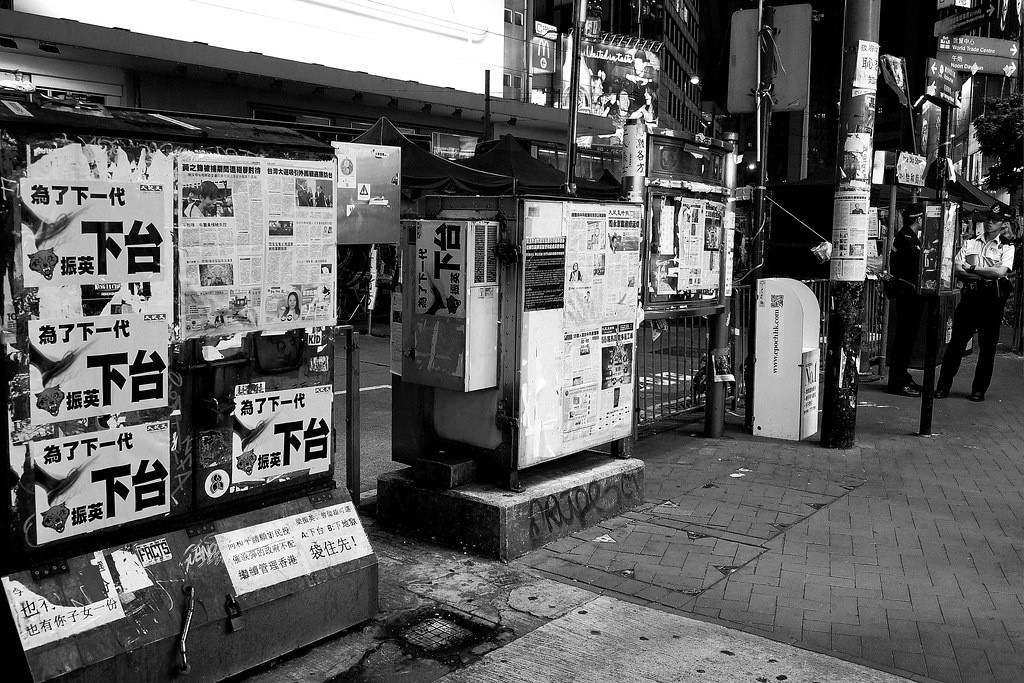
[907, 380, 923, 392]
[933, 388, 949, 398]
[970, 391, 985, 402]
[896, 386, 921, 397]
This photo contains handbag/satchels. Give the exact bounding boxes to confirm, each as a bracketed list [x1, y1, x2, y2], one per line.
[990, 276, 1013, 305]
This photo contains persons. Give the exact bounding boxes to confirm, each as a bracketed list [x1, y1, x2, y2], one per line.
[569, 262, 581, 281]
[281, 292, 301, 317]
[591, 49, 658, 129]
[183, 181, 333, 217]
[933, 202, 1015, 402]
[884, 203, 923, 397]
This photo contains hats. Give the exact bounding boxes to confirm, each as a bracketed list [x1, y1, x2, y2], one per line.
[902, 203, 924, 218]
[980, 202, 1016, 221]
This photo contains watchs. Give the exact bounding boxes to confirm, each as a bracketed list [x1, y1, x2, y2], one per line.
[970, 265, 975, 271]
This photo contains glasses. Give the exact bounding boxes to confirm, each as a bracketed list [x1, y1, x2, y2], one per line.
[986, 217, 999, 222]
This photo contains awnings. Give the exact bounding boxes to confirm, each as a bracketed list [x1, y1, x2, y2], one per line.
[953, 171, 1003, 206]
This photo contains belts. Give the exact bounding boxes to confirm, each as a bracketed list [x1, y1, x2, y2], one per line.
[963, 282, 990, 291]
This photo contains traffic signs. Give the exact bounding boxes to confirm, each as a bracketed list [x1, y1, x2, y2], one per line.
[934, 33, 1018, 78]
[926, 58, 961, 108]
[934, 0, 997, 37]
